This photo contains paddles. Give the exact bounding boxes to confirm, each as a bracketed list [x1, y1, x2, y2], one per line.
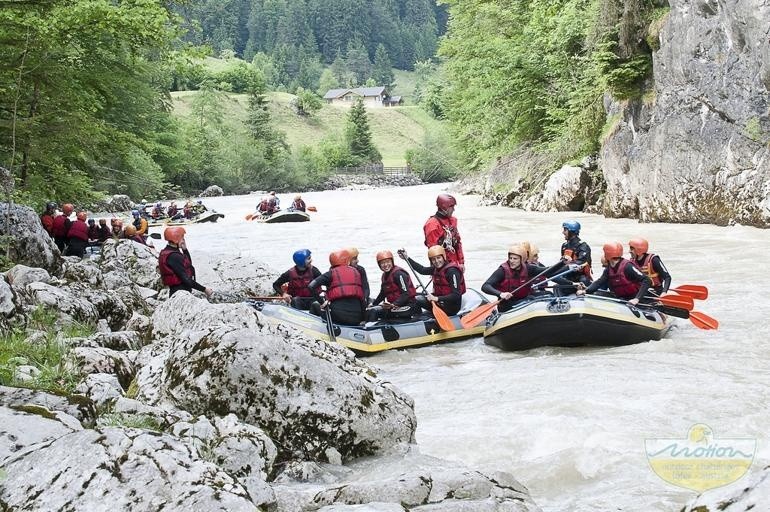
[304, 206, 317, 212]
[461, 259, 566, 329]
[247, 295, 326, 302]
[688, 311, 718, 329]
[596, 289, 693, 311]
[142, 233, 161, 239]
[666, 285, 708, 300]
[399, 249, 455, 331]
[585, 294, 689, 319]
[245, 211, 259, 220]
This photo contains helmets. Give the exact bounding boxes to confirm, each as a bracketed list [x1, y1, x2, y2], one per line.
[628, 237, 649, 256]
[88, 218, 137, 237]
[62, 203, 74, 214]
[46, 202, 57, 212]
[376, 249, 395, 266]
[132, 210, 139, 217]
[164, 226, 186, 244]
[141, 199, 202, 209]
[507, 240, 541, 264]
[603, 240, 624, 260]
[562, 219, 581, 235]
[292, 248, 312, 268]
[436, 194, 457, 211]
[77, 211, 87, 222]
[427, 245, 447, 265]
[328, 245, 359, 267]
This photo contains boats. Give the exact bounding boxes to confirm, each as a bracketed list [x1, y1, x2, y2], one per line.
[135, 210, 227, 227]
[259, 285, 492, 356]
[81, 234, 156, 258]
[251, 209, 311, 225]
[485, 289, 676, 354]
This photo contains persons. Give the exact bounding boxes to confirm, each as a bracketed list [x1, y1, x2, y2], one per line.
[273, 249, 321, 310]
[255, 190, 280, 218]
[423, 193, 466, 272]
[291, 195, 305, 212]
[309, 248, 366, 326]
[158, 225, 214, 299]
[398, 244, 466, 316]
[366, 250, 417, 322]
[41, 198, 208, 259]
[345, 247, 370, 305]
[480, 217, 670, 320]
